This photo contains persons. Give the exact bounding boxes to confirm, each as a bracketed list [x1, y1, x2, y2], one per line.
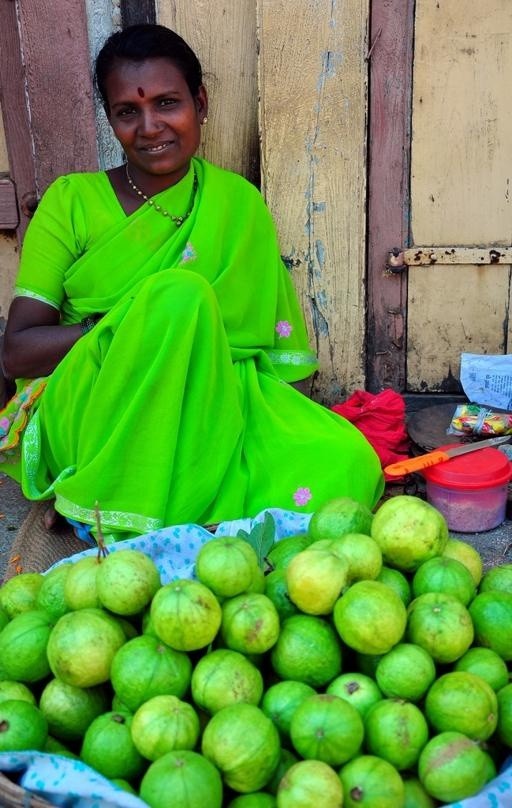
[0, 26, 387, 547]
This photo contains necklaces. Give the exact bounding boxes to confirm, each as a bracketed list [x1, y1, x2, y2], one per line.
[126, 163, 198, 227]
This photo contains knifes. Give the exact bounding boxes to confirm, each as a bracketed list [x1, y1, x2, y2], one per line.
[379, 436, 510, 479]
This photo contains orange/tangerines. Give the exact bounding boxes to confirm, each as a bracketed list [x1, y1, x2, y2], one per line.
[0, 492, 512, 808]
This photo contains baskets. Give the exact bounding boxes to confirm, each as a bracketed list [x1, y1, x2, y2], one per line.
[0, 501, 511, 802]
